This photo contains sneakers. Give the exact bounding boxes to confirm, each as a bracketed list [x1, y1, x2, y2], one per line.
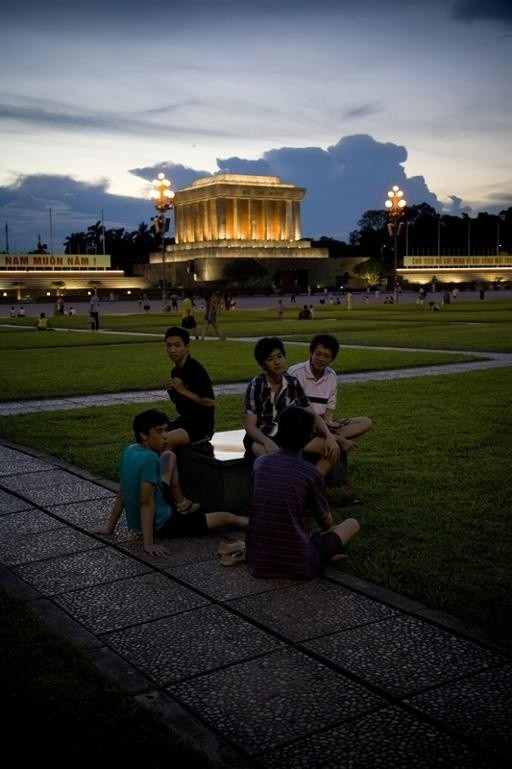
[179, 501, 200, 514]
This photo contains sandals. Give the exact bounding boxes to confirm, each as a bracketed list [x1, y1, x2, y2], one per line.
[218, 540, 246, 566]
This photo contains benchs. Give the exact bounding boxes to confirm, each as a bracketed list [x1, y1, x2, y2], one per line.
[174, 429, 255, 512]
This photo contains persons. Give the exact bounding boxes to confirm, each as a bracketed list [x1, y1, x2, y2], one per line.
[416, 283, 484, 312]
[170, 284, 237, 338]
[95, 408, 254, 563]
[152, 327, 217, 450]
[324, 285, 401, 311]
[246, 408, 365, 582]
[11, 291, 100, 331]
[237, 337, 342, 487]
[284, 333, 373, 451]
[278, 289, 315, 320]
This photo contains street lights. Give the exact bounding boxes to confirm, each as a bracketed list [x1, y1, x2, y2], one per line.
[150, 171, 176, 312]
[383, 185, 407, 305]
